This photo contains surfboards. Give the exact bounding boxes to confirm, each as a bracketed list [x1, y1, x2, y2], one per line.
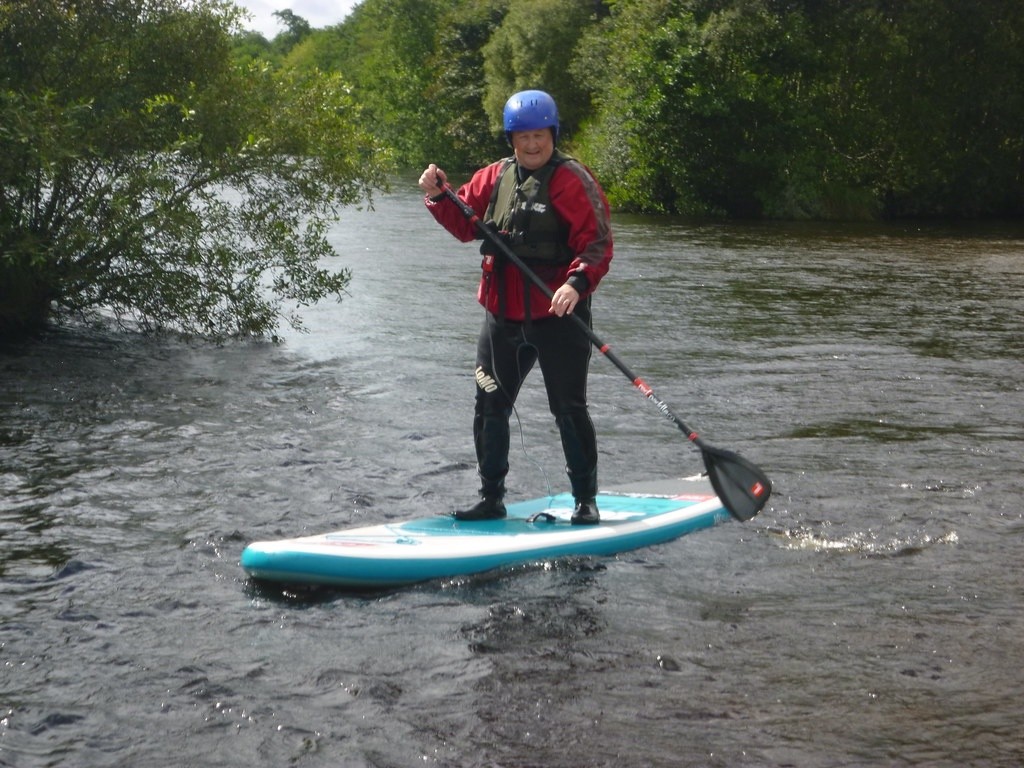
[241, 466, 731, 592]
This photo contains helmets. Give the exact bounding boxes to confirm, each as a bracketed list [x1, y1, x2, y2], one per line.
[503, 91, 559, 147]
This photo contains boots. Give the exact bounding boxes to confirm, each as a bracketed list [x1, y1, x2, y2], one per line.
[451, 462, 509, 520]
[565, 463, 600, 524]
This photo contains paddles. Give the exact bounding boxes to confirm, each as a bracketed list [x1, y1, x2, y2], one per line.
[432, 173, 773, 524]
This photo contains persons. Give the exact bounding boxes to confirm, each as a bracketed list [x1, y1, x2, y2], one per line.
[417, 91, 615, 526]
